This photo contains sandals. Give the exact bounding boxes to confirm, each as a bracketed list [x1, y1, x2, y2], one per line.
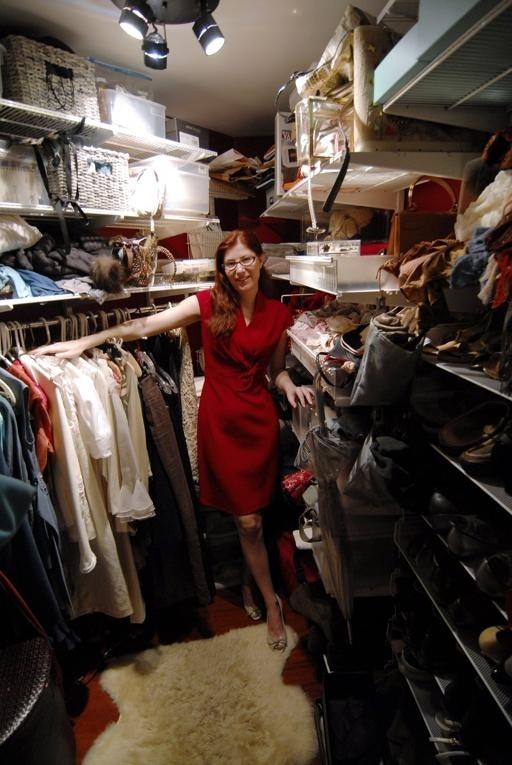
[267, 594, 287, 654]
[240, 588, 262, 620]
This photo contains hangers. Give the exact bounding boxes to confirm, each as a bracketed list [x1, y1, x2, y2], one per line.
[0, 301, 174, 408]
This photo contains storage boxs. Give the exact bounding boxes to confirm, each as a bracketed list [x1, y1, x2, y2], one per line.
[128, 154, 211, 216]
[104, 85, 168, 138]
[166, 117, 211, 149]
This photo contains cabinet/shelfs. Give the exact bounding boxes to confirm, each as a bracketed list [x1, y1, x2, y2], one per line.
[0, 95, 222, 313]
[261, 0, 512, 764]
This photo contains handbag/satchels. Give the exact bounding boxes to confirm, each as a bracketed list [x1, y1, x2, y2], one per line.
[295, 4, 403, 103]
[387, 210, 457, 256]
[458, 157, 512, 214]
[349, 315, 419, 408]
[107, 233, 158, 286]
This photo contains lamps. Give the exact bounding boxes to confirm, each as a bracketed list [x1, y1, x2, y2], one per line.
[112, 0, 226, 72]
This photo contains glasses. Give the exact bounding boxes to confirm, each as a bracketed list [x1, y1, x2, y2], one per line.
[224, 256, 258, 271]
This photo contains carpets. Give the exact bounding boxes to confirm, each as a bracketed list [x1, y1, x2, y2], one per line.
[76, 622, 318, 765]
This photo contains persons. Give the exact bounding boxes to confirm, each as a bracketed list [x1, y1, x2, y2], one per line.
[26, 229, 319, 653]
